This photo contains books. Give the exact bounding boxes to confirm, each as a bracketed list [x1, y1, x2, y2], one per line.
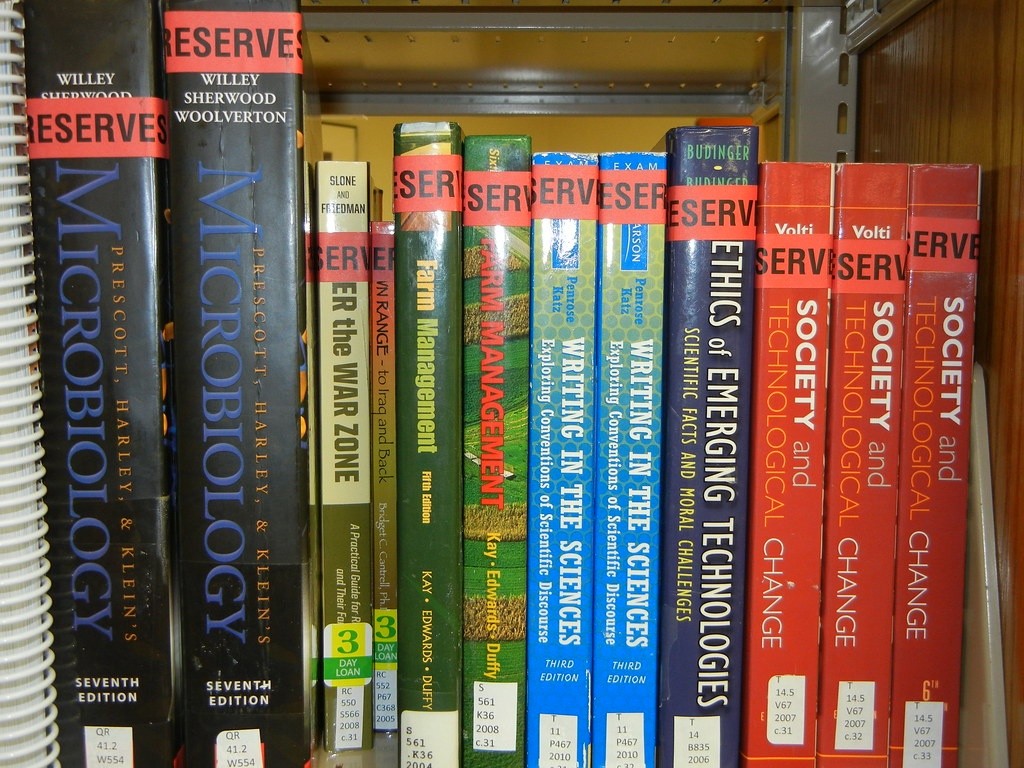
[0, 0, 1024, 768]
